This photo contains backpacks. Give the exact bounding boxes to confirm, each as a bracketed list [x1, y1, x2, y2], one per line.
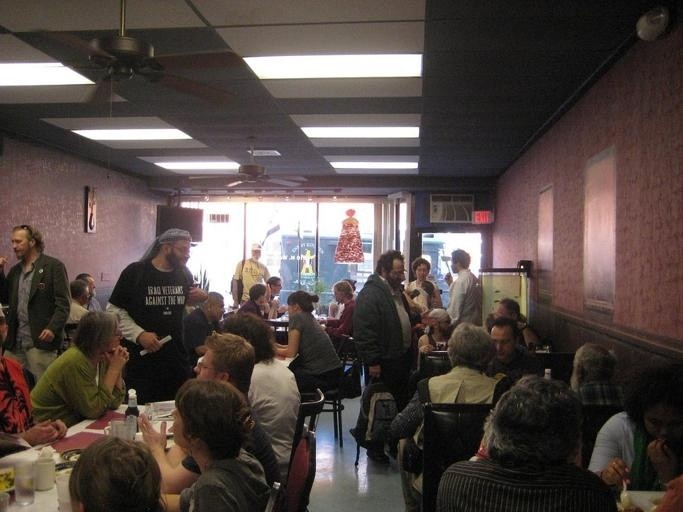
[349, 383, 402, 450]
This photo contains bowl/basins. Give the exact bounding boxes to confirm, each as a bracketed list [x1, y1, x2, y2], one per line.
[632, 491, 666, 511]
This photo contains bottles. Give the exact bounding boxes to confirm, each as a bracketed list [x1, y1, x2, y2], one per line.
[124, 388, 140, 434]
[544, 369, 551, 379]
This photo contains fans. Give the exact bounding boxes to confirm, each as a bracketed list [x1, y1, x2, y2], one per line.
[187, 137, 308, 188]
[37, 0, 243, 152]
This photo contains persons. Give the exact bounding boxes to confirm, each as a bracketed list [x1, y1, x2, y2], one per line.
[587, 365, 683, 495]
[390, 322, 498, 512]
[158, 379, 272, 512]
[0, 225, 71, 383]
[68, 279, 89, 321]
[318, 280, 356, 347]
[230, 242, 271, 309]
[264, 276, 289, 319]
[329, 279, 358, 319]
[76, 273, 102, 311]
[445, 249, 482, 327]
[405, 257, 443, 309]
[486, 298, 539, 349]
[225, 310, 301, 485]
[105, 228, 208, 404]
[490, 316, 542, 384]
[418, 308, 452, 352]
[435, 374, 618, 512]
[236, 283, 267, 316]
[353, 250, 414, 463]
[273, 291, 343, 390]
[1, 301, 68, 457]
[182, 292, 225, 369]
[69, 435, 168, 512]
[137, 332, 281, 494]
[30, 310, 129, 428]
[568, 344, 625, 470]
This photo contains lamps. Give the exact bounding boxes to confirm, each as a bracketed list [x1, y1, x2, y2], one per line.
[304, 189, 313, 202]
[226, 189, 233, 201]
[258, 190, 263, 201]
[201, 190, 209, 202]
[284, 190, 290, 202]
[332, 192, 338, 202]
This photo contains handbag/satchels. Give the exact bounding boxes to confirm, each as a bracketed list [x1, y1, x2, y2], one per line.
[229, 276, 244, 305]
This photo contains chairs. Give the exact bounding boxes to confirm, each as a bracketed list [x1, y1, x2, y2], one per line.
[264, 480, 285, 511]
[286, 388, 326, 483]
[302, 334, 357, 447]
[65, 322, 79, 348]
[276, 424, 317, 512]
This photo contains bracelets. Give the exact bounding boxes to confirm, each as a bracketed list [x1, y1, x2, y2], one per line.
[600, 471, 616, 488]
[520, 323, 532, 331]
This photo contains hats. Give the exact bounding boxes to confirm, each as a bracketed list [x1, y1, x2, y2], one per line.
[250, 243, 262, 251]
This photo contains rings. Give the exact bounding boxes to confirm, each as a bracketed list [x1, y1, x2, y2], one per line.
[612, 460, 616, 468]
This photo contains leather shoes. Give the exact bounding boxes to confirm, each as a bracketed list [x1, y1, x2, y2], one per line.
[388, 439, 396, 458]
[366, 446, 390, 464]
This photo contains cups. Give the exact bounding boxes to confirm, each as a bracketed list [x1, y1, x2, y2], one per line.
[56, 475, 70, 512]
[35, 458, 56, 489]
[14, 464, 35, 504]
[0, 492, 10, 512]
[104, 418, 137, 440]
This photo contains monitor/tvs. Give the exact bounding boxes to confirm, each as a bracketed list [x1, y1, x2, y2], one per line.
[155, 203, 204, 244]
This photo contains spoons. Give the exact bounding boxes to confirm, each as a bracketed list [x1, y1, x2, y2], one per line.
[620, 479, 631, 507]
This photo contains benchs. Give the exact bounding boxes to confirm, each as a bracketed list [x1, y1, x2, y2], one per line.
[419, 351, 577, 385]
[422, 403, 622, 511]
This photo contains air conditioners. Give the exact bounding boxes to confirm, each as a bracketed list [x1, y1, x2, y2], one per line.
[429, 193, 476, 224]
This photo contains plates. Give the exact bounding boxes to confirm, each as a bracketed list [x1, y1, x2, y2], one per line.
[60, 446, 85, 465]
